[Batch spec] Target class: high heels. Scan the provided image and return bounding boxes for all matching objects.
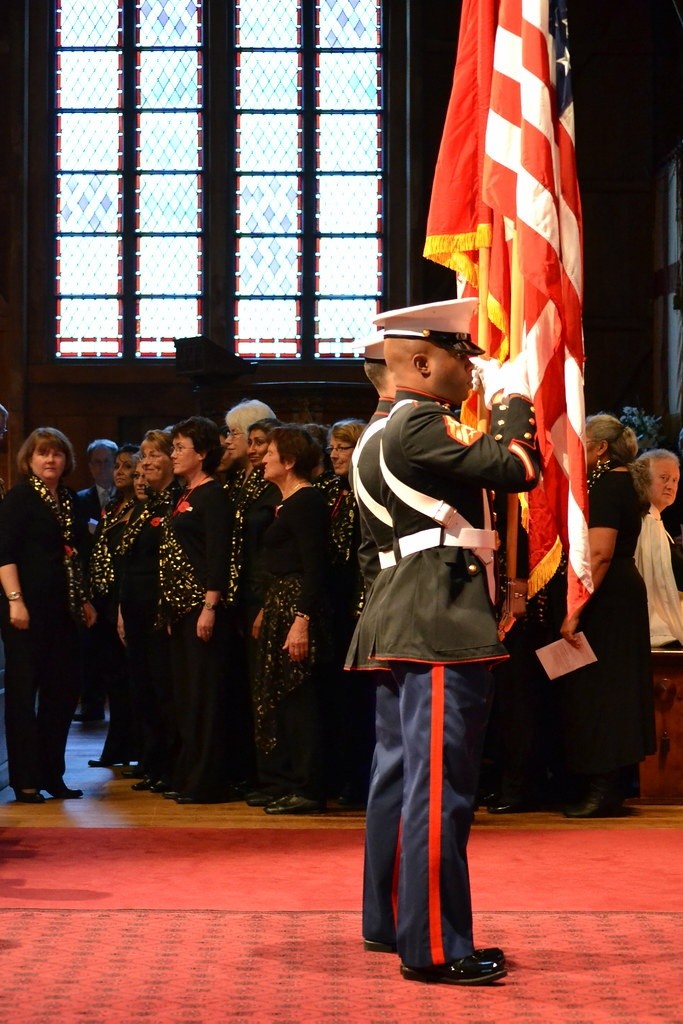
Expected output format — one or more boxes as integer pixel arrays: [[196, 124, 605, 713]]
[[88, 759, 130, 767], [12, 785, 44, 803], [38, 780, 83, 798]]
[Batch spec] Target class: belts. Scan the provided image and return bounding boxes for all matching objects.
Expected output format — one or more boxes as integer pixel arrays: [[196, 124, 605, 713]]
[[397, 527, 496, 559], [377, 549, 397, 570]]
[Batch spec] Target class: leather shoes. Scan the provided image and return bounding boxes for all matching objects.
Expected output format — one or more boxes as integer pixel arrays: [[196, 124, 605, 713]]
[[131, 774, 172, 794], [565, 780, 625, 818], [400, 948, 504, 975], [121, 764, 147, 778], [243, 789, 289, 806], [337, 783, 368, 807], [475, 785, 534, 815], [164, 786, 208, 804], [265, 793, 326, 815], [403, 954, 507, 985]]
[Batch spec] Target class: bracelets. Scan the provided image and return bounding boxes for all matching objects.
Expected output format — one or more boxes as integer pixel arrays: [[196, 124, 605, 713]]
[[295, 612, 309, 621], [514, 591, 526, 598]]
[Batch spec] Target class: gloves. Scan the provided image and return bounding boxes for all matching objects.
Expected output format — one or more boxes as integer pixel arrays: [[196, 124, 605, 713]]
[[469, 356, 504, 410], [499, 351, 535, 402]]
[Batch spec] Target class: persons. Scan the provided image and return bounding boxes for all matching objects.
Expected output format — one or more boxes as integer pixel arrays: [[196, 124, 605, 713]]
[[78, 398, 278, 803], [342, 296, 543, 985], [229, 418, 367, 812], [478, 409, 683, 819], [0, 426, 97, 802]]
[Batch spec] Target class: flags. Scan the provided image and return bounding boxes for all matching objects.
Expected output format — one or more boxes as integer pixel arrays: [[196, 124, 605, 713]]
[[423, 0, 594, 621]]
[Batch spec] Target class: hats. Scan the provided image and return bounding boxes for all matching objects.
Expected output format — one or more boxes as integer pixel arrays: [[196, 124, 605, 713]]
[[366, 330, 386, 360], [373, 295, 486, 355]]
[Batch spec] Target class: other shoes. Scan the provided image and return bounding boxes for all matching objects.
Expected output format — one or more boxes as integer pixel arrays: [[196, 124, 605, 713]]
[[225, 778, 257, 800], [73, 711, 105, 722], [363, 935, 397, 953]]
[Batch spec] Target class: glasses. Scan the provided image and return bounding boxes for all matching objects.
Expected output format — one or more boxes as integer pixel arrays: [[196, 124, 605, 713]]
[[0, 428, 8, 440], [226, 432, 246, 439], [170, 445, 195, 454], [326, 446, 354, 454]]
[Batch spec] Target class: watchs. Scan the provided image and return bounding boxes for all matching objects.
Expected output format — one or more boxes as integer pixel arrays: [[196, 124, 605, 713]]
[[204, 602, 216, 610], [7, 591, 23, 601]]
